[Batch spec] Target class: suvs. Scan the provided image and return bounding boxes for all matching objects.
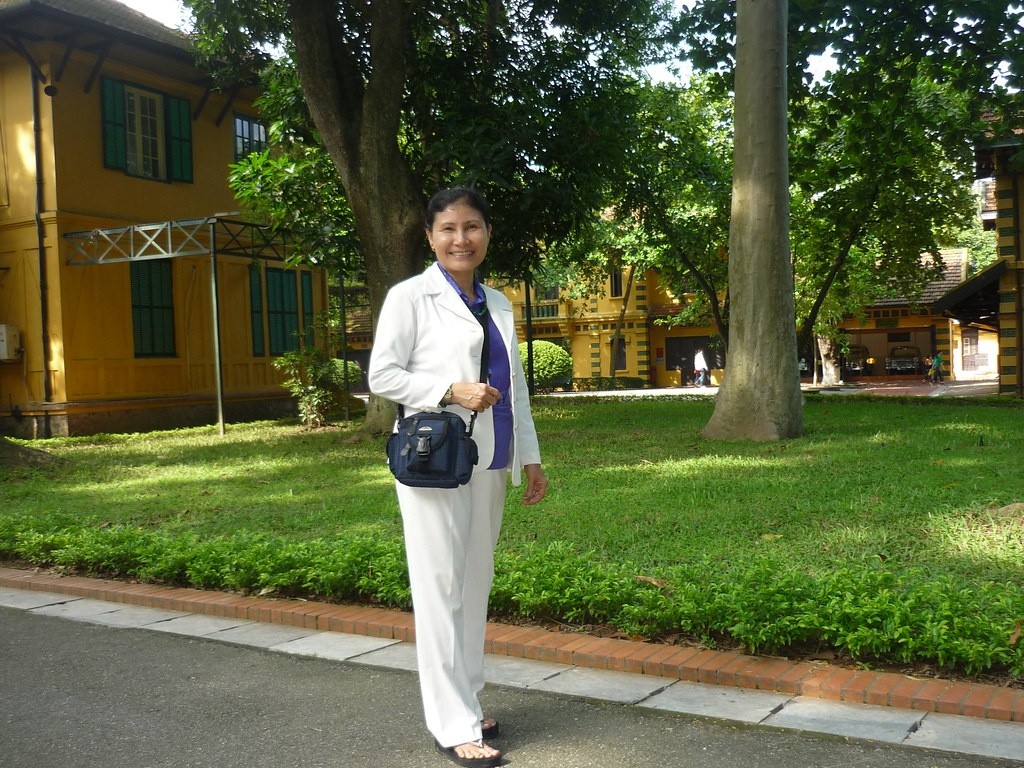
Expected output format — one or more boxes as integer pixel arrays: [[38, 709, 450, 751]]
[[886, 345, 923, 375], [839, 344, 873, 377]]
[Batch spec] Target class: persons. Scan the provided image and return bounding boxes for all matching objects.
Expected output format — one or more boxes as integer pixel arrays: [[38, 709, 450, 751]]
[[694, 348, 708, 387], [929, 351, 946, 384], [367, 189, 549, 768]]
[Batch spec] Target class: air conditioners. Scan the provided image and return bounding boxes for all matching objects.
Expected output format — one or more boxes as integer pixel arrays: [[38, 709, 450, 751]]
[[0, 323, 22, 360]]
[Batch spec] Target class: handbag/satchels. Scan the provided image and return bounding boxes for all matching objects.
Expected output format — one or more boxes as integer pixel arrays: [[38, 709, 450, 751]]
[[386, 411, 479, 488]]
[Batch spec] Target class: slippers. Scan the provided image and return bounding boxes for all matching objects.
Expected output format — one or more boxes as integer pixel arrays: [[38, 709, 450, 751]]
[[478, 713, 499, 740], [434, 734, 501, 767]]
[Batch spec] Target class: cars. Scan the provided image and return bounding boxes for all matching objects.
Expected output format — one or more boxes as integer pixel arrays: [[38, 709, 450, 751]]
[[797, 353, 815, 379]]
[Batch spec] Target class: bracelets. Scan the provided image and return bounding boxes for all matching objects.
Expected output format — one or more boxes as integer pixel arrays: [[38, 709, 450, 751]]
[[443, 387, 451, 405]]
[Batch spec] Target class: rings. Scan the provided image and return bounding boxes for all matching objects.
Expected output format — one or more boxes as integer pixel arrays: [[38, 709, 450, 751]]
[[533, 490, 537, 493]]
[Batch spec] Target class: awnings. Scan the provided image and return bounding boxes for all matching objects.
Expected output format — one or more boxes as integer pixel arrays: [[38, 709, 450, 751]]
[[929, 258, 1007, 331]]
